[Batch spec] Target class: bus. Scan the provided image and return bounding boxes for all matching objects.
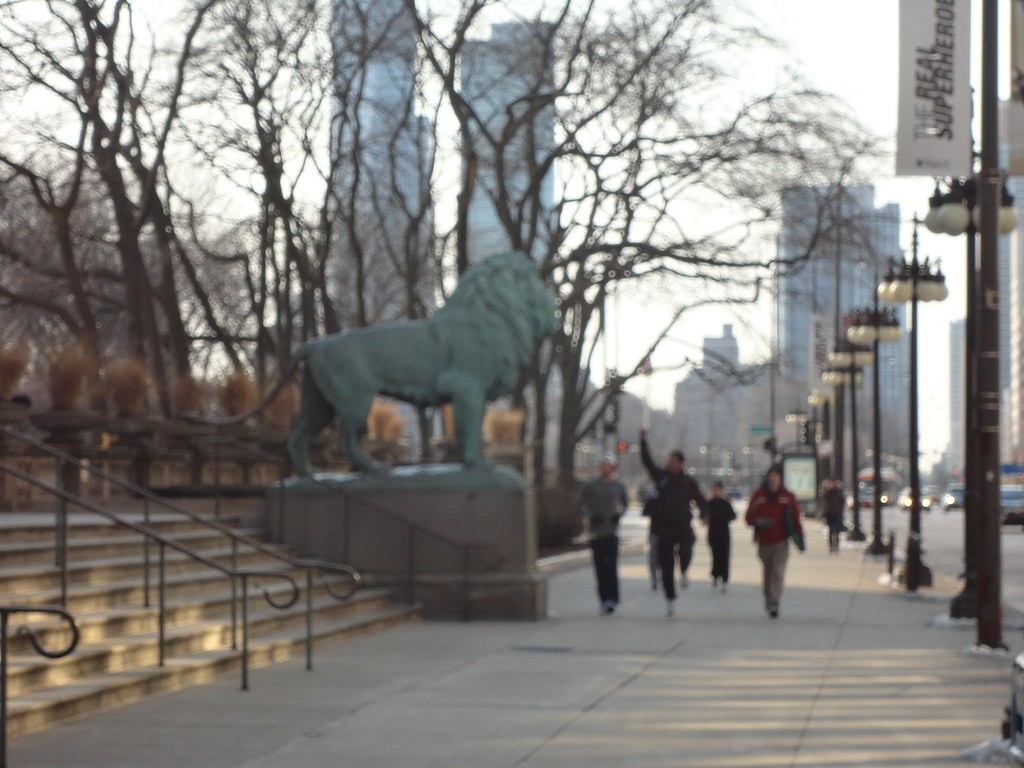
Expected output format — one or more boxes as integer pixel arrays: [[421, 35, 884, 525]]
[[857, 466, 903, 508]]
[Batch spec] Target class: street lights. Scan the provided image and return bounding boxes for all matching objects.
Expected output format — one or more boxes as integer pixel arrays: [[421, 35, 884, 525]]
[[922, 172, 1023, 618], [786, 335, 873, 536], [844, 281, 903, 557], [875, 210, 950, 591]]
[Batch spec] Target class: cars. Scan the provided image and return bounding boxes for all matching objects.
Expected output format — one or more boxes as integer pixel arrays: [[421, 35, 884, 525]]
[[942, 486, 967, 511], [998, 484, 1024, 526], [898, 485, 934, 512]]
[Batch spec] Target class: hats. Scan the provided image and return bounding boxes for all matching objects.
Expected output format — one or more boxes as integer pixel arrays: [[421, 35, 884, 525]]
[[599, 452, 619, 470]]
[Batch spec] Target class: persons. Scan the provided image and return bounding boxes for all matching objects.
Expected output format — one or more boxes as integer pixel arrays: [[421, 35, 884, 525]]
[[638, 429, 709, 602], [579, 452, 630, 613], [820, 478, 846, 553], [746, 466, 805, 617], [700, 482, 736, 593]]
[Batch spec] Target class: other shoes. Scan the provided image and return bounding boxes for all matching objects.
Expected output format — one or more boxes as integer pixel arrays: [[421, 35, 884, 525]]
[[603, 601, 615, 614], [768, 604, 778, 617]]
